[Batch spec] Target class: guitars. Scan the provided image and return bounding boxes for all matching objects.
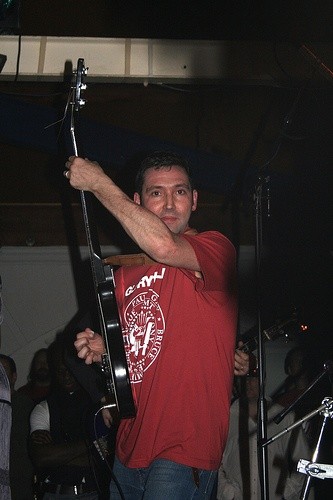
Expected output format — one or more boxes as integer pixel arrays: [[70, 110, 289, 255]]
[[69, 58, 136, 419], [94, 308, 301, 461]]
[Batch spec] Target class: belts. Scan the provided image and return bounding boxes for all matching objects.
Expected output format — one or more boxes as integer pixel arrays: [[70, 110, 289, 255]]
[[43, 483, 97, 495]]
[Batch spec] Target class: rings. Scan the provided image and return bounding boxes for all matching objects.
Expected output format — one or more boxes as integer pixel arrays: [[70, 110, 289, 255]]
[[64, 170, 70, 177]]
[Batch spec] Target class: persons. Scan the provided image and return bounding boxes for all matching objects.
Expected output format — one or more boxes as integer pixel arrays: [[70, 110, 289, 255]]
[[276, 348, 318, 407], [74, 327, 250, 377], [0, 344, 314, 500], [218, 364, 316, 500], [62, 152, 237, 500]]
